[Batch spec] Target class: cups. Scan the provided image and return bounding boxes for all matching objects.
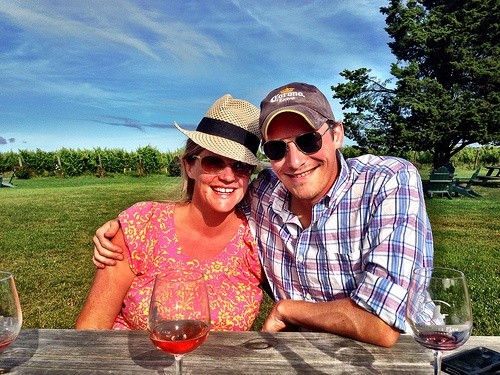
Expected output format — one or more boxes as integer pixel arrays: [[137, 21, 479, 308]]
[[0, 270, 22, 351]]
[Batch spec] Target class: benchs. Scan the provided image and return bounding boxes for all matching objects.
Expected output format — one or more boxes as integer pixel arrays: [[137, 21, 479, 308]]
[[478, 175, 500, 183]]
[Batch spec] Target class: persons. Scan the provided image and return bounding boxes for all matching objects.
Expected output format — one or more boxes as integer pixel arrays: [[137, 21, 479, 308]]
[[91, 83, 445, 348], [75, 93, 266, 334]]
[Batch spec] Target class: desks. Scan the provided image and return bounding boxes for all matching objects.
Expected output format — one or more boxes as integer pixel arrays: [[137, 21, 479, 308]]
[[0, 328, 500, 375], [484, 166, 500, 176]]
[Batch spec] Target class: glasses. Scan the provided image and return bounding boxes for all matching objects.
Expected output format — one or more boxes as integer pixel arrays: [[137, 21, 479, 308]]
[[191, 154, 256, 178], [263, 124, 334, 162]]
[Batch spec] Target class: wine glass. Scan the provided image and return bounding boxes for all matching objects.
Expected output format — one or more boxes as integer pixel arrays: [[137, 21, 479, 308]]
[[146, 269, 211, 375], [406, 267, 472, 375]]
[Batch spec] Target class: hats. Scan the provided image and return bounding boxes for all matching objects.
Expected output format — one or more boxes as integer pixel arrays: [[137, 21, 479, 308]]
[[259, 82, 335, 141], [172, 94, 263, 172]]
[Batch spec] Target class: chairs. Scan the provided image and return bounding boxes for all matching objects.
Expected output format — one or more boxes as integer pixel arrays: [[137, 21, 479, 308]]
[[454, 166, 482, 198], [428, 166, 452, 199]]
